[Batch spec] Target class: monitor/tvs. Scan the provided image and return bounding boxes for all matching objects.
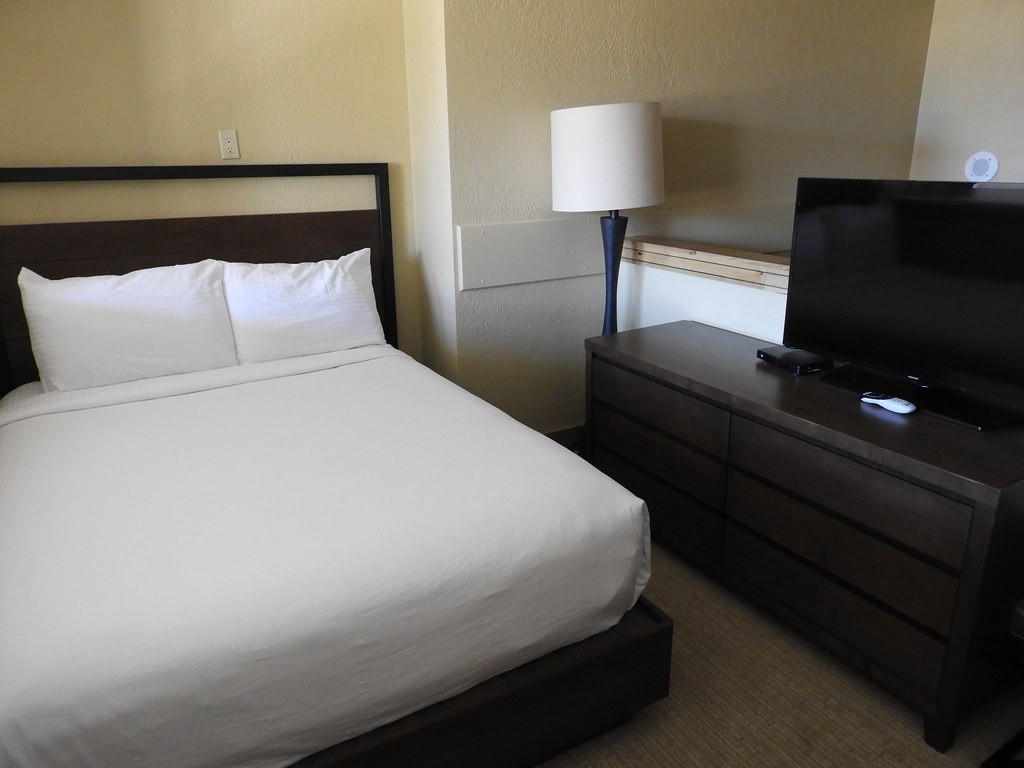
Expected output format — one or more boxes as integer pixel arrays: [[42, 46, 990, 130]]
[[782, 178, 1023, 429]]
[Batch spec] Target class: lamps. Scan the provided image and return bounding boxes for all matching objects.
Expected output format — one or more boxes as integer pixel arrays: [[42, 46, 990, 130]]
[[551, 102, 665, 335]]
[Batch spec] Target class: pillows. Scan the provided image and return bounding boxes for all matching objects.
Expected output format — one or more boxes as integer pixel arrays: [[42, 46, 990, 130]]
[[219, 248, 386, 366], [18, 258, 239, 393]]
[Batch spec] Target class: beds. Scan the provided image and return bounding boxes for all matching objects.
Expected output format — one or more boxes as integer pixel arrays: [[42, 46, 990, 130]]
[[1, 163, 674, 768]]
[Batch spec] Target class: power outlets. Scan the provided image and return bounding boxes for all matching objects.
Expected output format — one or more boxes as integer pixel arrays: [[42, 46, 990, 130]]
[[219, 130, 240, 160]]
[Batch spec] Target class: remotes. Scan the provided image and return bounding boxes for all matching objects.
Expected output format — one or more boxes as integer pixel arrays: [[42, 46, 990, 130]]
[[860, 390, 915, 414]]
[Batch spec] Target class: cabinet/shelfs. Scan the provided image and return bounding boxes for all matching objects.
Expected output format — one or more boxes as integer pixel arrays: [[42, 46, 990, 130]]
[[585, 320, 1024, 755]]
